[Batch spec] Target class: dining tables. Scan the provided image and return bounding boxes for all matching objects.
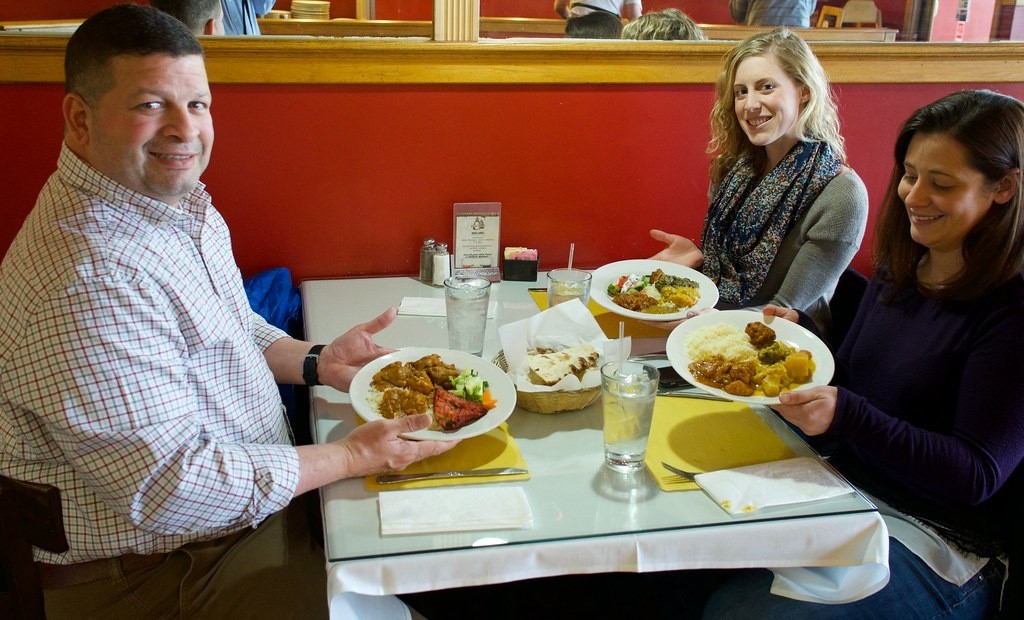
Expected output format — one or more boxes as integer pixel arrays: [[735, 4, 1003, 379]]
[[299, 269, 890, 620]]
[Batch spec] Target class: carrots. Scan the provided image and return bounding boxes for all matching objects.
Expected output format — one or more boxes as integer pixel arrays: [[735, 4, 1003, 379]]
[[481, 389, 497, 409]]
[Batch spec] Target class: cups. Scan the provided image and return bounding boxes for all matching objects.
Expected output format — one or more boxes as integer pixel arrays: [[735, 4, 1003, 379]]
[[547, 268, 593, 308], [443, 274, 491, 359], [600, 360, 660, 473]]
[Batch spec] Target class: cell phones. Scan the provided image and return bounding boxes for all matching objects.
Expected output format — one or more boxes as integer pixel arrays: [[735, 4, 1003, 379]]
[[656, 366, 691, 389]]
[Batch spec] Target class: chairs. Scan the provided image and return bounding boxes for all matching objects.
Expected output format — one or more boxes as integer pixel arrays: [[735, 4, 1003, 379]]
[[0, 475, 70, 620]]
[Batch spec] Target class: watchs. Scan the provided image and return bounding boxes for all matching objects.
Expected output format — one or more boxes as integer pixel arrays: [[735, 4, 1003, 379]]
[[303, 345, 326, 386]]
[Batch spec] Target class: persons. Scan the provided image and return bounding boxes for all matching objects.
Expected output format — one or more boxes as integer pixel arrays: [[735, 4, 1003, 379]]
[[221, 0, 276, 35], [621, 8, 706, 42], [565, 12, 623, 39], [149, 1, 225, 35], [0, 6, 464, 620], [730, 0, 817, 29], [555, 0, 643, 20], [705, 89, 1020, 619], [646, 26, 869, 310]]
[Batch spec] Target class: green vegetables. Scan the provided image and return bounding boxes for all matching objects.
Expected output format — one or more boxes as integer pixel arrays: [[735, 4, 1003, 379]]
[[448, 368, 489, 402]]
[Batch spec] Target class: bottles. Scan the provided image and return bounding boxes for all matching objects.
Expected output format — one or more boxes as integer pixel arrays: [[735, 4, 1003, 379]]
[[432, 242, 450, 288], [418, 237, 435, 281]]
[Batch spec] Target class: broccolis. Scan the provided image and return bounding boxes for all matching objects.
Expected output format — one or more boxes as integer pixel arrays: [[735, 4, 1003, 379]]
[[608, 276, 651, 294]]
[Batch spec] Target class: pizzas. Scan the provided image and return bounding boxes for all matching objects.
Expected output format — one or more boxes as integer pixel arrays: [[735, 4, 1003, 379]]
[[434, 388, 487, 428]]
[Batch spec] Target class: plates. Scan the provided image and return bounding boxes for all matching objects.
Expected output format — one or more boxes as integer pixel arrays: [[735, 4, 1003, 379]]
[[589, 259, 719, 321], [665, 310, 835, 405], [349, 347, 517, 443], [290, 0, 331, 20]]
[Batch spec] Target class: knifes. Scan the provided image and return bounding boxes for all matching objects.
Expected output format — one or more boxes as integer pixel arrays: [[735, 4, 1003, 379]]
[[377, 467, 528, 485], [656, 389, 734, 402], [529, 287, 547, 292]]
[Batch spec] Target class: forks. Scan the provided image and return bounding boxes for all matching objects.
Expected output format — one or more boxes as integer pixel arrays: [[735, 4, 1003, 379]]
[[660, 460, 704, 480]]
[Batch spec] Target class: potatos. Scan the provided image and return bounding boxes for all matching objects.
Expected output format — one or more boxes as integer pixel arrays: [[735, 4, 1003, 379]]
[[753, 352, 810, 395]]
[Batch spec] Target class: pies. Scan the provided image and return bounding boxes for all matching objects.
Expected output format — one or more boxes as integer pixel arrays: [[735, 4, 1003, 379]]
[[526, 343, 600, 385]]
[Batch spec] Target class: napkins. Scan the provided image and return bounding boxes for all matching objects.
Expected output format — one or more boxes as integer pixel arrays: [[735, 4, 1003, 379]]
[[378, 486, 534, 536], [694, 455, 856, 518], [398, 295, 497, 320]]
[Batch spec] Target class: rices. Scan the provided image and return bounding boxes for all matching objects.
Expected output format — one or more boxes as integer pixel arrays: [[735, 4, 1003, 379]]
[[685, 323, 757, 363], [644, 284, 662, 300]]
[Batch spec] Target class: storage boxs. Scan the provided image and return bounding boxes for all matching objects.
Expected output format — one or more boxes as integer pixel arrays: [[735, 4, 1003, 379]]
[[501, 254, 541, 283]]
